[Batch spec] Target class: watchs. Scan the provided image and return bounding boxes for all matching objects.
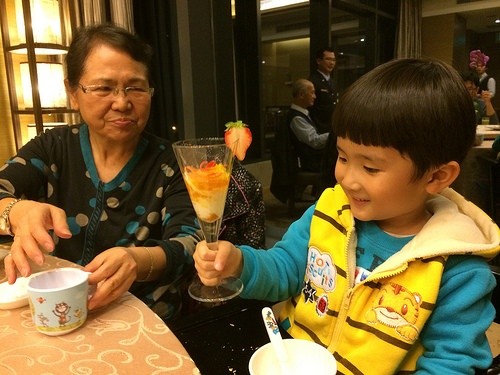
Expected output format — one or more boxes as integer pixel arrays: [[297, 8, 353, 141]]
[[0, 198, 23, 237]]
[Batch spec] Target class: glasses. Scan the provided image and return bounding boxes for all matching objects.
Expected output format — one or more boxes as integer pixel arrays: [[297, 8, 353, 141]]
[[76, 82, 154, 99]]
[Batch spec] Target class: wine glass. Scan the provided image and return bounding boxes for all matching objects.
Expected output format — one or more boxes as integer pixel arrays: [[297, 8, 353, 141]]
[[172, 138, 244, 302]]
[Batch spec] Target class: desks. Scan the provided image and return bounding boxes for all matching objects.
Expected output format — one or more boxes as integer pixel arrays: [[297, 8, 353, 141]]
[[449, 123, 500, 221], [0, 242, 202, 375]]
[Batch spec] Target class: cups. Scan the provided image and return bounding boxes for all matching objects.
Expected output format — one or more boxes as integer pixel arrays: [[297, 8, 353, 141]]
[[25, 267, 107, 336], [481, 117, 489, 125], [248, 339, 338, 375]]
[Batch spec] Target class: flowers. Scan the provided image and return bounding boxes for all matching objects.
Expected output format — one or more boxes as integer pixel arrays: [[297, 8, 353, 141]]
[[469, 48, 489, 72]]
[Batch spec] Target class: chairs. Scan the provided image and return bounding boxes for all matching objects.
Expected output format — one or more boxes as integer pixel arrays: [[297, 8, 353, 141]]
[[475, 152, 500, 223], [270, 111, 325, 218]]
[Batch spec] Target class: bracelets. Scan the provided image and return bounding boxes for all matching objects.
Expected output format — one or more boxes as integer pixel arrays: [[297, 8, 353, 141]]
[[136, 246, 153, 283]]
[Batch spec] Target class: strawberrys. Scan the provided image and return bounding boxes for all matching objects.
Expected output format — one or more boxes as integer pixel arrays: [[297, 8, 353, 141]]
[[224, 120, 252, 161]]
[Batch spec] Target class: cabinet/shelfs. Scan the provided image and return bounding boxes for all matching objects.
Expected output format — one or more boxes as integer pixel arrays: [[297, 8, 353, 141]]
[[0, 0, 81, 154]]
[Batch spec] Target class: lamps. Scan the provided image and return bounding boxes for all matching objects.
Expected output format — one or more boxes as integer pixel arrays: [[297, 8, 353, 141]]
[[4, 0, 69, 55], [28, 123, 68, 143], [19, 61, 68, 109]]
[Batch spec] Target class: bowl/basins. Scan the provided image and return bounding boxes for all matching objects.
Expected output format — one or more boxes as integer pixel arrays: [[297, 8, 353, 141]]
[[474, 133, 484, 146]]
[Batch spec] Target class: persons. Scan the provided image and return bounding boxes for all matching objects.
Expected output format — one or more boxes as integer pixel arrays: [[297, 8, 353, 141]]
[[284, 78, 332, 174], [194, 57, 500, 375], [461, 50, 499, 124], [0, 25, 213, 332], [309, 47, 340, 135]]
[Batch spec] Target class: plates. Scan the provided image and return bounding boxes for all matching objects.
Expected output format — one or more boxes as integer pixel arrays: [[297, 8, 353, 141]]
[[0, 277, 29, 310], [476, 124, 500, 139]]
